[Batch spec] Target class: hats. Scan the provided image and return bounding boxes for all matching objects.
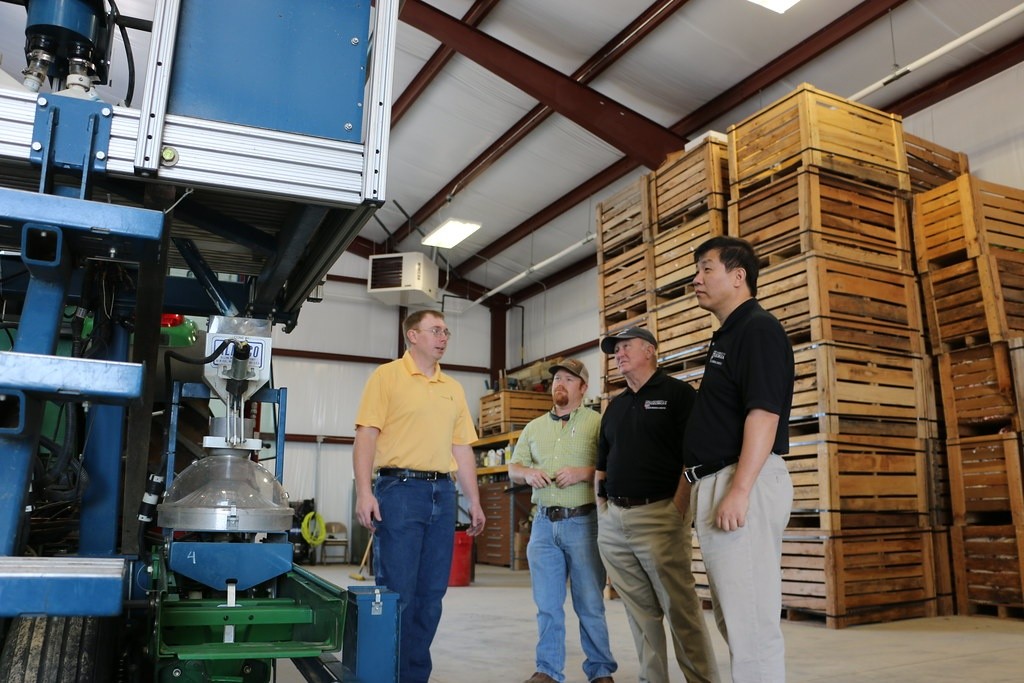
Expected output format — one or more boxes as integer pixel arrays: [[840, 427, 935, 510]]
[[547, 359, 591, 384], [600, 327, 659, 351]]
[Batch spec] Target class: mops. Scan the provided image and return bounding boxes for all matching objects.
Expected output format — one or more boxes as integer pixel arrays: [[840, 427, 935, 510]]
[[349, 532, 374, 581]]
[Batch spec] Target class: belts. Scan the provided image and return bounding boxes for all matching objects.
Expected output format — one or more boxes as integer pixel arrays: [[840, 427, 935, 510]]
[[541, 503, 596, 521], [378, 467, 449, 480], [681, 457, 742, 484], [607, 496, 669, 510]]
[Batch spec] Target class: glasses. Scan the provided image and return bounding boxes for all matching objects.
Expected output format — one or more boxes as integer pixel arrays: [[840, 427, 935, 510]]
[[414, 329, 453, 340], [548, 412, 574, 422]]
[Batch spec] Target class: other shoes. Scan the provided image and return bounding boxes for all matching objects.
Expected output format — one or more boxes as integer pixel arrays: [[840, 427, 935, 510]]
[[527, 671, 560, 683], [590, 676, 614, 683]]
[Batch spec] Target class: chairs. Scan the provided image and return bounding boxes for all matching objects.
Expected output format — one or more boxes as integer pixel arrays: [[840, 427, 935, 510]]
[[320, 522, 350, 567]]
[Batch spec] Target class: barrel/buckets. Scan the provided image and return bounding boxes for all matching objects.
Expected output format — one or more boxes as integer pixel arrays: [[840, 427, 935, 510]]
[[447, 532, 472, 586]]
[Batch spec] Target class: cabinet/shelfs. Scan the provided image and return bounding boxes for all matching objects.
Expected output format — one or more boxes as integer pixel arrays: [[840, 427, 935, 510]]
[[470, 429, 534, 571]]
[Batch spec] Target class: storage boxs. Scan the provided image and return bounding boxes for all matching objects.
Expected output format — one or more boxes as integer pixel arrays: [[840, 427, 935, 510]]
[[513, 531, 529, 571], [597, 81, 1024, 630], [479, 390, 554, 438]]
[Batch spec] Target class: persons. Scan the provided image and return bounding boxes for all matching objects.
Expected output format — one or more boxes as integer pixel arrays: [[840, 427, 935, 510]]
[[508, 357, 619, 683], [594, 325, 722, 683], [681, 237, 794, 683], [352, 309, 487, 683]]
